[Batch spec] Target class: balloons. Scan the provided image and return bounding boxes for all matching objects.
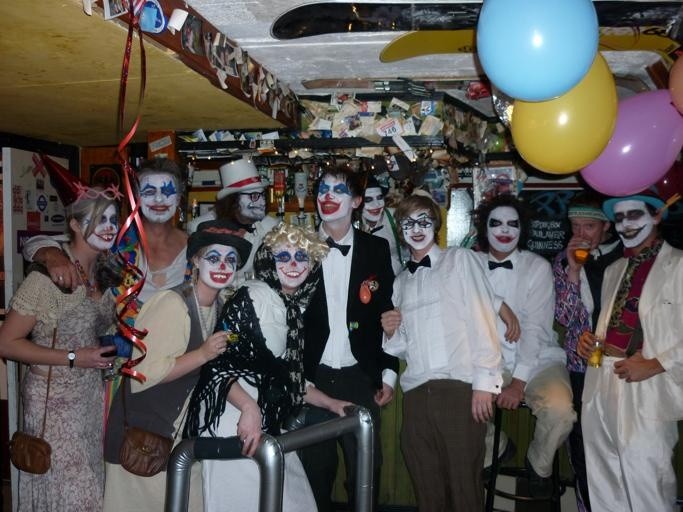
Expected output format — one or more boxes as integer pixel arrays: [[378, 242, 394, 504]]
[[576, 88, 682, 198], [474, 1, 599, 104], [669, 54, 682, 116], [509, 51, 618, 177]]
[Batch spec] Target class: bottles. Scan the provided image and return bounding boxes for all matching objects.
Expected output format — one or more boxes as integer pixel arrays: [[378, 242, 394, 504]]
[[294, 168, 309, 221], [257, 164, 269, 195], [272, 168, 288, 219]]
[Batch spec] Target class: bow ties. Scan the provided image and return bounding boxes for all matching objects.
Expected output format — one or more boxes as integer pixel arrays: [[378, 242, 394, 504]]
[[488, 260, 512, 270], [406, 255, 431, 274], [326, 236, 350, 256]]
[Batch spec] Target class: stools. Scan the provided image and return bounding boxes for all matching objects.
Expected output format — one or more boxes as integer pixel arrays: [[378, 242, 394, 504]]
[[483, 402, 581, 512]]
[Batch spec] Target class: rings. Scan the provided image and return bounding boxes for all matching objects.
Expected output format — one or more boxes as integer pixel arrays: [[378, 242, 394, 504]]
[[58, 275, 63, 281]]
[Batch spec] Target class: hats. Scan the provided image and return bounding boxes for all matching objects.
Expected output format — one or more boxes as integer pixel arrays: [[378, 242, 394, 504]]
[[358, 171, 389, 198], [602, 184, 668, 223], [216, 158, 270, 200], [36, 151, 88, 208], [186, 220, 252, 271]]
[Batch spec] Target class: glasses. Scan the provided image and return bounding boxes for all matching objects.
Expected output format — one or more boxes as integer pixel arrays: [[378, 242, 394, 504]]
[[243, 190, 270, 202], [73, 182, 122, 202], [399, 217, 431, 230]]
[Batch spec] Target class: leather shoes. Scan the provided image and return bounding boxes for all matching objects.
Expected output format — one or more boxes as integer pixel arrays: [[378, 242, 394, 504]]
[[482, 437, 515, 482], [524, 454, 553, 500]]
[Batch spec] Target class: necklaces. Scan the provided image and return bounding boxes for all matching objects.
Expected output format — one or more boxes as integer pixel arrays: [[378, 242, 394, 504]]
[[190, 288, 217, 339]]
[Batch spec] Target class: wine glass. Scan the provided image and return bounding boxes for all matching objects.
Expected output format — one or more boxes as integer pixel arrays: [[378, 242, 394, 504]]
[[97, 334, 123, 383]]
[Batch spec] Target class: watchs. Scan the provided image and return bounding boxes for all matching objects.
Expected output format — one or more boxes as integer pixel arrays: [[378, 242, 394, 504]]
[[67, 350, 75, 369]]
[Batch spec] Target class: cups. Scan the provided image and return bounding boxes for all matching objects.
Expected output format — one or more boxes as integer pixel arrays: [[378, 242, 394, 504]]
[[572, 237, 590, 265], [587, 331, 605, 369]]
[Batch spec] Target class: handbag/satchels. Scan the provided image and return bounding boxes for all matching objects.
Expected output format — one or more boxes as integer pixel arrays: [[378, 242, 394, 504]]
[[10, 432, 51, 474], [120, 429, 174, 476]]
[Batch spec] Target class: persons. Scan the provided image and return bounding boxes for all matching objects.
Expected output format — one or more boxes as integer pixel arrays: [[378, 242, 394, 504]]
[[102, 218, 252, 511], [379, 189, 503, 512], [171, 221, 356, 512], [465, 193, 578, 501], [352, 174, 412, 276], [180, 159, 280, 286], [19, 157, 190, 431], [0, 186, 123, 512], [294, 165, 399, 512], [552, 188, 624, 512], [581, 183, 683, 512]]
